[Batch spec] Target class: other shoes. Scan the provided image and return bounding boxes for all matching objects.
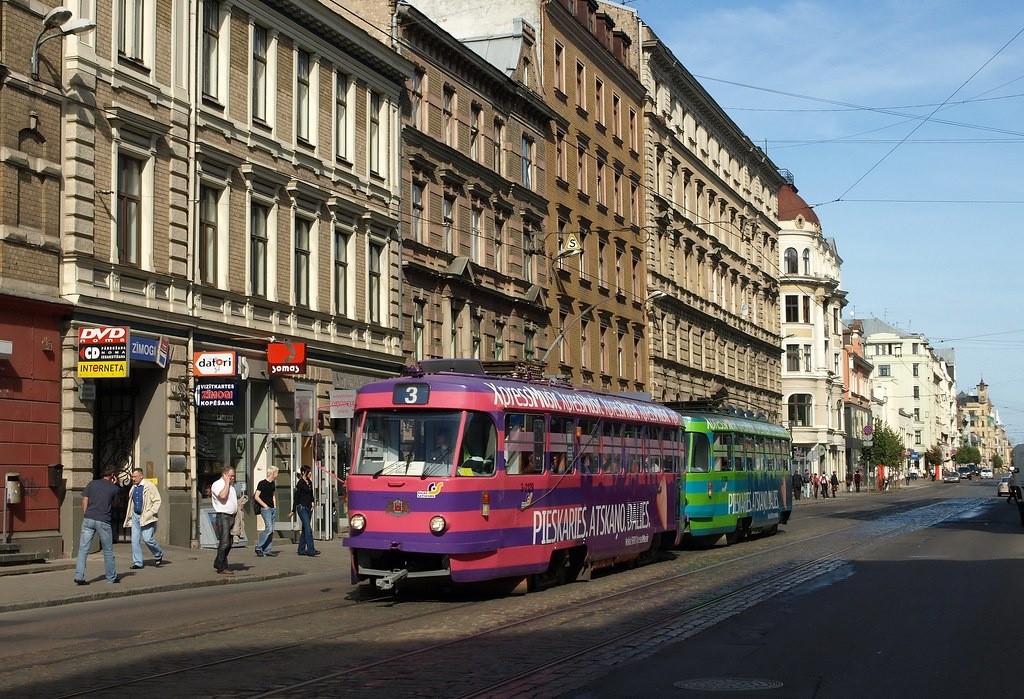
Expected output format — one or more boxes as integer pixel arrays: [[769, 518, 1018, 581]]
[[113, 579, 120, 583], [74, 579, 89, 585], [155, 557, 162, 566], [130, 565, 144, 569], [298, 550, 321, 556], [214, 565, 235, 575], [255, 549, 277, 558]]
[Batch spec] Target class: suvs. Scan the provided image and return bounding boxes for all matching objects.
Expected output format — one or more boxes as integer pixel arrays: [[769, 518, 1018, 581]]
[[957, 467, 973, 481]]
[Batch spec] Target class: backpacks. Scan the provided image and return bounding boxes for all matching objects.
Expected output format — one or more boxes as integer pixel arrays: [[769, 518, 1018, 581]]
[[821, 476, 827, 485]]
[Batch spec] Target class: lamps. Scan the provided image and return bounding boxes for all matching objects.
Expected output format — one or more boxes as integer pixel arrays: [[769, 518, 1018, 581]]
[[642, 291, 662, 311], [36, 18, 98, 74], [33, 6, 72, 74], [653, 291, 667, 306], [780, 332, 797, 341]]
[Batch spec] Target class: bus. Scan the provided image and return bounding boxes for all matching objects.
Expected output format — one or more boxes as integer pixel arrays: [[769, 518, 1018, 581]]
[[341, 358, 685, 598], [658, 399, 795, 546]]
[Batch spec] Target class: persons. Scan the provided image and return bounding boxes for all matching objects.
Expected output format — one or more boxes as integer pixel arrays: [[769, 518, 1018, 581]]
[[846, 470, 861, 492], [74, 465, 120, 585], [426, 426, 474, 477], [287, 464, 321, 556], [792, 469, 839, 500], [1007, 471, 1019, 505], [583, 453, 659, 473], [211, 466, 238, 574], [255, 466, 279, 557], [123, 468, 163, 569], [889, 465, 934, 487]]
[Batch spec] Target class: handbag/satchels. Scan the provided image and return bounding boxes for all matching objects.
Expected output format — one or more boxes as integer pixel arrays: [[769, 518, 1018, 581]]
[[252, 493, 261, 515]]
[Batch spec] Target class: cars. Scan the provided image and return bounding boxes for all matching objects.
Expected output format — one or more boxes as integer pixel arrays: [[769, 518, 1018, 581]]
[[944, 472, 961, 483], [980, 469, 994, 479], [997, 476, 1017, 498]]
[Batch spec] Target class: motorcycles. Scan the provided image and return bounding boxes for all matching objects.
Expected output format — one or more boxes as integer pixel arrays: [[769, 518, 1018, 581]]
[[975, 469, 979, 476]]
